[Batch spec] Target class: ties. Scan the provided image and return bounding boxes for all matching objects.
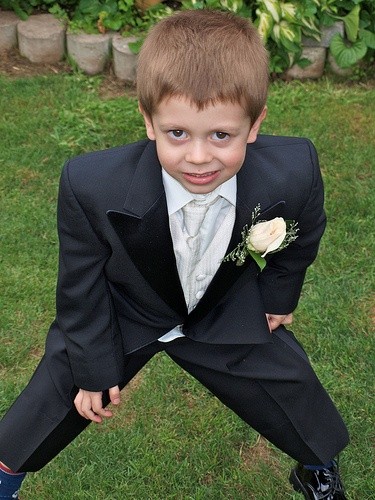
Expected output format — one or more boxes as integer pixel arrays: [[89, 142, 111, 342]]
[[181, 200, 207, 258]]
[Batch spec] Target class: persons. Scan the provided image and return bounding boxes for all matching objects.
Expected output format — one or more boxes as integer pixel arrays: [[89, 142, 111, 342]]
[[0, 7, 350, 500]]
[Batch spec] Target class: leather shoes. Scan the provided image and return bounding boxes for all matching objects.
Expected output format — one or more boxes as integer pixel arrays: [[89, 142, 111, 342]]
[[289, 465, 348, 499]]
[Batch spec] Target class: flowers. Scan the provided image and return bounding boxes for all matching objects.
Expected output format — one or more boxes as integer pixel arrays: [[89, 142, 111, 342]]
[[217, 202, 299, 272]]
